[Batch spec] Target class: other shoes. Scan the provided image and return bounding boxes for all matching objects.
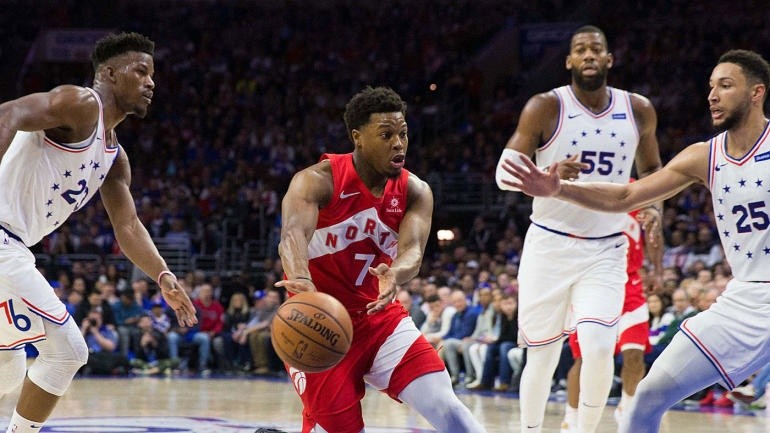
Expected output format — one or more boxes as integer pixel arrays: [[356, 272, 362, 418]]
[[129, 360, 285, 378], [452, 373, 770, 433]]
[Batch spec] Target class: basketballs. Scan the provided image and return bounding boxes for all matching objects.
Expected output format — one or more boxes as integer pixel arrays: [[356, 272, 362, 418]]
[[271, 292, 353, 373]]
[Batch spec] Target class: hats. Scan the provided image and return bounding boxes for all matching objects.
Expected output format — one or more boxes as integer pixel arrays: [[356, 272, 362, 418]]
[[151, 300, 164, 307], [467, 261, 478, 269], [252, 290, 266, 299]]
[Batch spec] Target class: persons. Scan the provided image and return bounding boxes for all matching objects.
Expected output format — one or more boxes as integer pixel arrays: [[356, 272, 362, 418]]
[[495, 46, 770, 433], [0, 30, 202, 433], [558, 176, 653, 433], [250, 84, 491, 433], [156, 271, 177, 289], [490, 22, 668, 433], [0, 0, 770, 410]]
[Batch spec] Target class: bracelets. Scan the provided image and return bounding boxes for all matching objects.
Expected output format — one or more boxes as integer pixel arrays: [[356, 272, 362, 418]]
[[290, 275, 314, 281]]
